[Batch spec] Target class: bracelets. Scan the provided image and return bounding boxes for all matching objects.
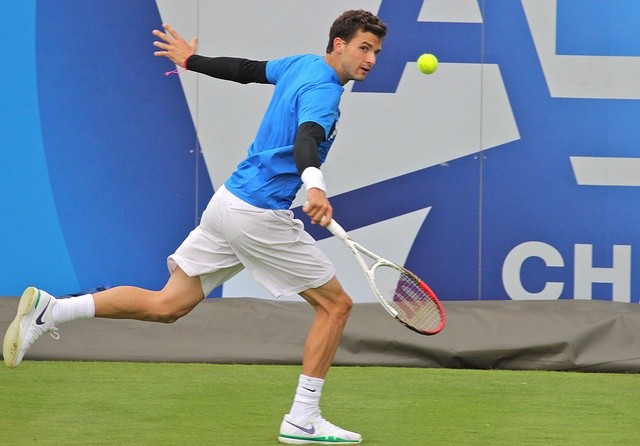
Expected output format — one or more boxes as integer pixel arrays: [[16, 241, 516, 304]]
[[184, 54, 194, 70]]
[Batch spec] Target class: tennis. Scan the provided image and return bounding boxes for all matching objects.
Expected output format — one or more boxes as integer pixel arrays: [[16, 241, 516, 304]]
[[416, 53, 438, 74]]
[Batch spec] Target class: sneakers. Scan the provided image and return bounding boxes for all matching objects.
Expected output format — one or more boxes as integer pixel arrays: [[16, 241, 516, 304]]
[[278, 413, 363, 445], [2, 286, 60, 369]]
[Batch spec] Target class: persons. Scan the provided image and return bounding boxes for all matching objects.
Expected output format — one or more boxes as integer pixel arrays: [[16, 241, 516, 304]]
[[2, 10, 386, 445]]
